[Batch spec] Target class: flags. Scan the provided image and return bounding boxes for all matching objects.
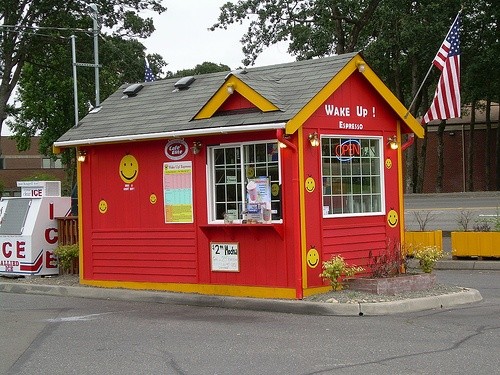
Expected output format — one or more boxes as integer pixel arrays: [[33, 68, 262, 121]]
[[144, 65, 156, 82], [419, 12, 462, 124]]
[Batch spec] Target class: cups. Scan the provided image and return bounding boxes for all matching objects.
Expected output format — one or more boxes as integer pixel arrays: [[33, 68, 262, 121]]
[[247, 181, 257, 201]]
